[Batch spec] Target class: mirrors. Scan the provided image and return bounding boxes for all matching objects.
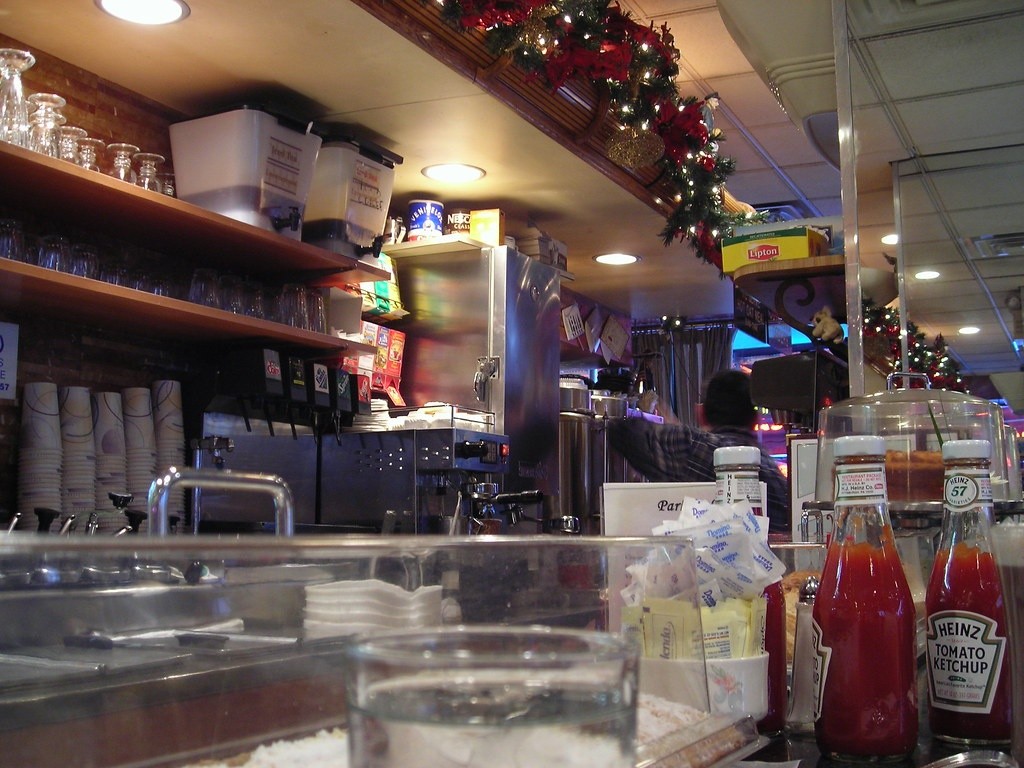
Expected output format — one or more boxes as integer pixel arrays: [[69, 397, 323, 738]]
[[830, 1, 1023, 502]]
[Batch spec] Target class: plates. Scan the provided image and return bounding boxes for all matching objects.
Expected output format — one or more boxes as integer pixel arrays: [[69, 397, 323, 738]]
[[301, 577, 443, 642]]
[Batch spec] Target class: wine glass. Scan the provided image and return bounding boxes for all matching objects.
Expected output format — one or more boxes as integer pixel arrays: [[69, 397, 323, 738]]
[[0, 47, 176, 198]]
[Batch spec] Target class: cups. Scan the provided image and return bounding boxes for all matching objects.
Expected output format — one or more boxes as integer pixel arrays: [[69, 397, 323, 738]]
[[504, 235, 519, 251], [0, 215, 327, 335], [469, 518, 503, 534], [15, 379, 186, 538], [338, 625, 643, 768], [987, 519, 1024, 768]]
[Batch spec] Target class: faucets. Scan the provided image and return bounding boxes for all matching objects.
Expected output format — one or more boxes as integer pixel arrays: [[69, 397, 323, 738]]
[[191, 435, 235, 534]]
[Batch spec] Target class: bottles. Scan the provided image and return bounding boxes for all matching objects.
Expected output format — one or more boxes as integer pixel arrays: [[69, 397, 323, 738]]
[[370, 509, 420, 592], [785, 600, 818, 744], [711, 444, 788, 730], [383, 215, 408, 246], [811, 433, 921, 767], [924, 438, 1012, 753]]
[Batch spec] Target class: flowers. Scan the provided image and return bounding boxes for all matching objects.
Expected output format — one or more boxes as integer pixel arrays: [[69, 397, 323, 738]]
[[429, 1, 976, 394]]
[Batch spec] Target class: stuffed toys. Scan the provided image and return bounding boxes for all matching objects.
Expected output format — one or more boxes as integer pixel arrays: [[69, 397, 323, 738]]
[[811, 307, 844, 344]]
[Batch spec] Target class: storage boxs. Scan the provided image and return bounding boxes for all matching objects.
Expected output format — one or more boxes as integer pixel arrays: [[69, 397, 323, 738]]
[[720, 225, 829, 276], [469, 208, 505, 246]]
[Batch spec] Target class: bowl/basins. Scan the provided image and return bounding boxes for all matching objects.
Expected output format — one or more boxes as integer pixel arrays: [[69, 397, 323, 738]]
[[645, 650, 770, 727]]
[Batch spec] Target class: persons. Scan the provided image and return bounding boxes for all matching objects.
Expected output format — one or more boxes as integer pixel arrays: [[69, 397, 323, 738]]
[[608, 370, 788, 533], [701, 91, 722, 132]]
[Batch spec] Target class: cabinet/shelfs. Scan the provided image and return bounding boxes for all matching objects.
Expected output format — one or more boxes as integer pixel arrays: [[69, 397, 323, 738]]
[[0, 140, 393, 360]]
